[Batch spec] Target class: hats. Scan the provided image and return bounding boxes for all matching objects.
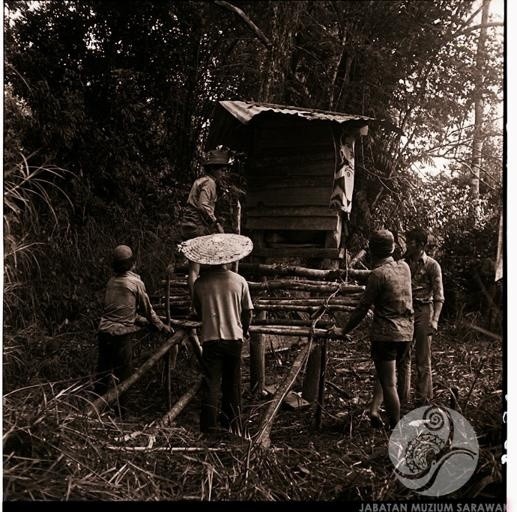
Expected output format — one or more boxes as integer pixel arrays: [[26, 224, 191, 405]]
[[202, 151, 231, 166], [179, 233, 256, 266], [113, 245, 132, 262], [370, 229, 397, 247]]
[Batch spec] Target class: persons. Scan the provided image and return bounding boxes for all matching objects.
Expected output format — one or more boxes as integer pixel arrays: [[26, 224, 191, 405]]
[[403, 228, 445, 407], [177, 233, 255, 433], [94, 244, 175, 399], [327, 230, 414, 429], [181, 151, 231, 297]]
